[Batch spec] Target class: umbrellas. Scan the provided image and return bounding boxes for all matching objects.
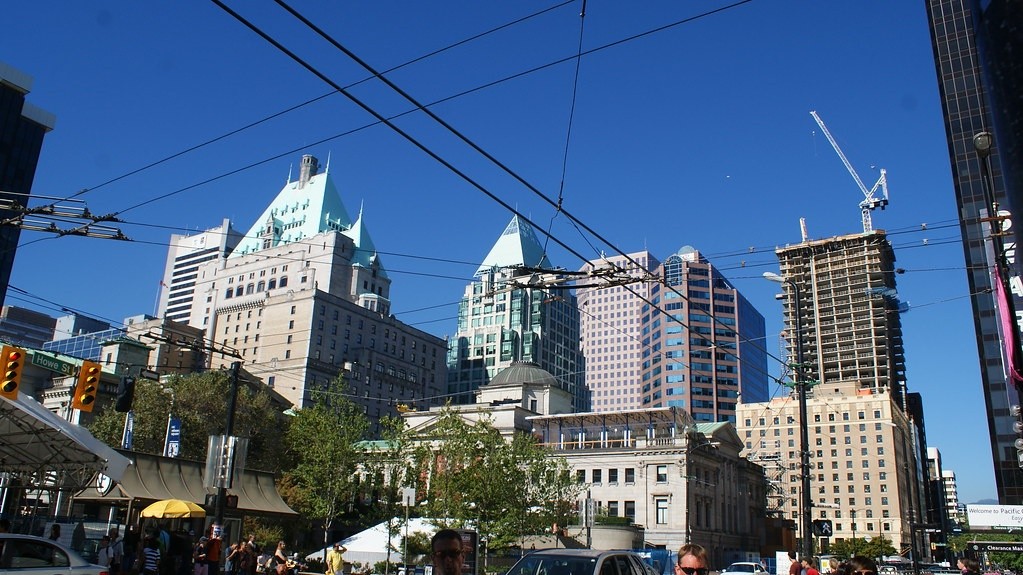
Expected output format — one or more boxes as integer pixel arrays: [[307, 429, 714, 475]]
[[140, 499, 206, 531]]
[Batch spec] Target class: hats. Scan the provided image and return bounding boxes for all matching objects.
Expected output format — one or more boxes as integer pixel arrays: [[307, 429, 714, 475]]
[[199, 536, 209, 543]]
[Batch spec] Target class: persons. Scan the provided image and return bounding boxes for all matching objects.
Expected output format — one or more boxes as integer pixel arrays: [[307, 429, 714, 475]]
[[759, 549, 880, 575], [431, 529, 466, 575], [0, 518, 14, 568], [49, 524, 61, 559], [97, 525, 302, 575], [326, 541, 347, 575], [674, 542, 711, 575], [956, 557, 981, 575]]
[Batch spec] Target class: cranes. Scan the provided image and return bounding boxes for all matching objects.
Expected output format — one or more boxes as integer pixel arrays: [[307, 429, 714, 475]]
[[810, 111, 889, 232]]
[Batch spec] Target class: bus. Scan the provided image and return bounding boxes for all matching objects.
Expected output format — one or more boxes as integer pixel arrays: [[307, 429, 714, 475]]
[[883, 556, 914, 575]]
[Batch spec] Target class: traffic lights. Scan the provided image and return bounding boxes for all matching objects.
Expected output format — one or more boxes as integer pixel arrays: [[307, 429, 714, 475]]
[[205, 494, 218, 507], [72, 361, 102, 412], [814, 520, 832, 536], [226, 494, 239, 509], [0, 345, 26, 400]]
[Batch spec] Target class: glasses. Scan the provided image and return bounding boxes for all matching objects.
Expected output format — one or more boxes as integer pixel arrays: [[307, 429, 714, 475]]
[[678, 564, 709, 575], [850, 570, 875, 575], [432, 549, 464, 558]]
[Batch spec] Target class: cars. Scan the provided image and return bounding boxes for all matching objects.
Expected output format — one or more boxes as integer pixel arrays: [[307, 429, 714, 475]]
[[505, 548, 649, 575], [928, 566, 943, 573], [0, 533, 110, 575], [719, 562, 770, 575]]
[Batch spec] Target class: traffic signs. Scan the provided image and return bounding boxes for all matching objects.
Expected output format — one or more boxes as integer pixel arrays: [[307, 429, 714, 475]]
[[32, 351, 74, 376]]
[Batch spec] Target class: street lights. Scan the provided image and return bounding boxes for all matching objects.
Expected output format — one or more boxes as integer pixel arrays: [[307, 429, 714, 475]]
[[762, 272, 813, 558], [881, 421, 920, 575], [852, 508, 870, 557], [685, 442, 721, 544], [879, 519, 895, 566]]
